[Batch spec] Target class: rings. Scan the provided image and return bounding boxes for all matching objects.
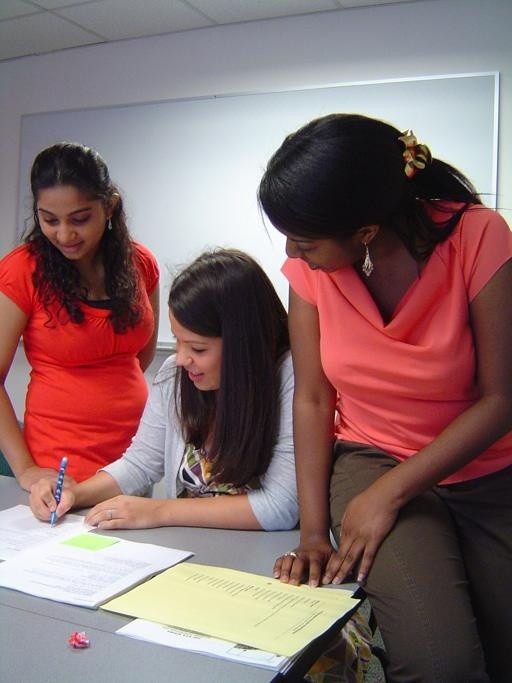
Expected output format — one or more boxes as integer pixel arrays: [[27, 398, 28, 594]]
[[284, 551, 297, 557], [108, 509, 113, 520]]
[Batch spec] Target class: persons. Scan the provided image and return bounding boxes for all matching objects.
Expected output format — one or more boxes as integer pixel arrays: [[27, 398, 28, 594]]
[[28, 249, 299, 531], [0, 141, 159, 492], [256, 114, 512, 683]]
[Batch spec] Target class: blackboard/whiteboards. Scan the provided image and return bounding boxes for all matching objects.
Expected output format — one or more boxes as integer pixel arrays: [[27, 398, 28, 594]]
[[17, 70, 503, 353]]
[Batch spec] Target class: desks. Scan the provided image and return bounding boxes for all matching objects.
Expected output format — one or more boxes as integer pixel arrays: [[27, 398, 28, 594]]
[[0, 473, 369, 682]]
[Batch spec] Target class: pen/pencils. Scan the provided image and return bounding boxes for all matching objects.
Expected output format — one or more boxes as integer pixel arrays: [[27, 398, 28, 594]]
[[49, 454, 68, 527]]
